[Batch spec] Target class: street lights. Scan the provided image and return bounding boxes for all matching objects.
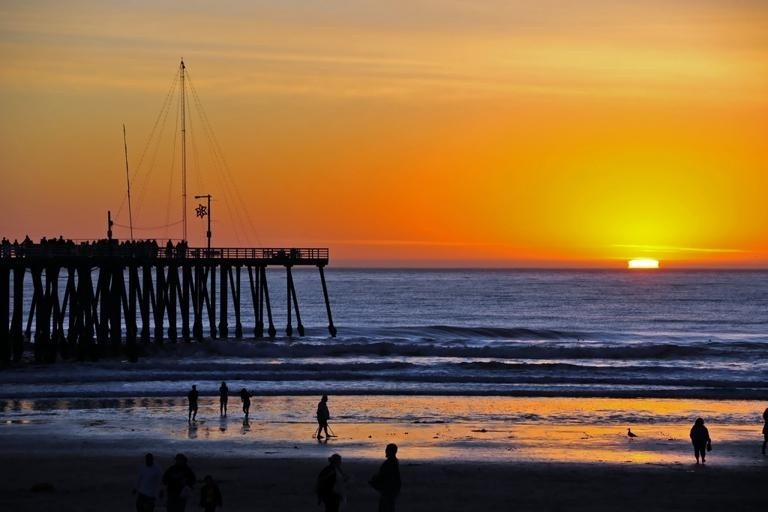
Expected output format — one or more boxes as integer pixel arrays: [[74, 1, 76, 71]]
[[195, 195, 210, 247]]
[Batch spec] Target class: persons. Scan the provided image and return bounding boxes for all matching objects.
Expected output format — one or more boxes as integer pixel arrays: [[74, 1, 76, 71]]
[[315, 453, 351, 510], [130, 452, 163, 512], [239, 414, 251, 435], [689, 417, 712, 465], [186, 384, 199, 423], [161, 453, 196, 512], [198, 473, 225, 512], [218, 380, 230, 419], [239, 387, 254, 417], [187, 420, 198, 439], [762, 407, 768, 455], [370, 443, 403, 512], [316, 394, 331, 439], [0, 233, 189, 260], [218, 414, 228, 433]]
